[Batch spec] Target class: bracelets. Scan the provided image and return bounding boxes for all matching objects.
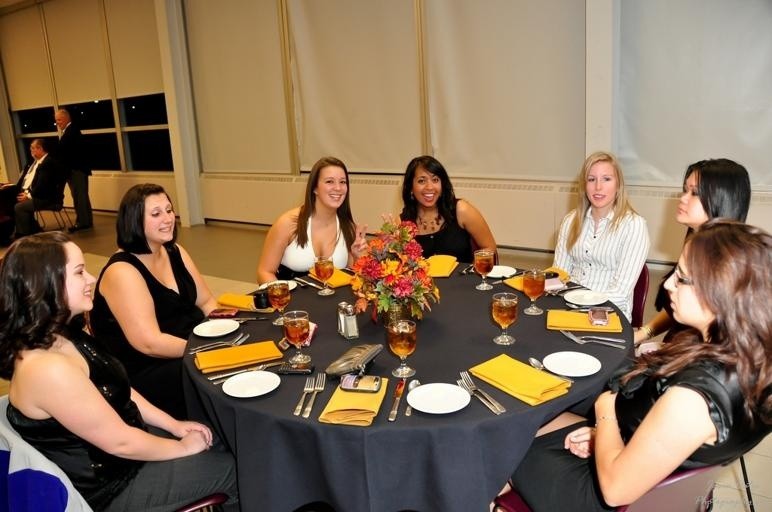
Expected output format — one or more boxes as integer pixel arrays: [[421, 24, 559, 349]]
[[640, 323, 654, 340], [591, 413, 619, 431]]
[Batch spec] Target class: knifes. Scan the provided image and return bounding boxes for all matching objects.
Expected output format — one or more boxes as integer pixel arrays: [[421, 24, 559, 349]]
[[387, 378, 407, 421], [208, 361, 284, 381], [491, 270, 525, 285], [294, 276, 323, 290]]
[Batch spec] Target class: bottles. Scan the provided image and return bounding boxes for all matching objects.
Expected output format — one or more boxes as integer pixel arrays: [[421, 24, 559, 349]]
[[337, 301, 347, 336], [345, 303, 360, 339]]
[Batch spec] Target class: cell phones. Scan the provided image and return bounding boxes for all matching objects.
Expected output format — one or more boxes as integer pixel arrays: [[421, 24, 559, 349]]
[[280, 361, 314, 375], [589, 309, 609, 326], [341, 375, 382, 392]]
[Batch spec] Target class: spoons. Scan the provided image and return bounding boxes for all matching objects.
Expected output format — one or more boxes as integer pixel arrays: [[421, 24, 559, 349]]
[[566, 303, 611, 309], [212, 364, 270, 386], [528, 357, 574, 383], [405, 379, 421, 417]]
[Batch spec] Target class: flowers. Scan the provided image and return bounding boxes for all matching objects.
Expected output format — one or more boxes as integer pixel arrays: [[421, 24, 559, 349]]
[[345, 220, 441, 324]]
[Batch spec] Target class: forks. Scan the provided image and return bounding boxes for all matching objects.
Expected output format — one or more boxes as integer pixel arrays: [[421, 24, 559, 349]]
[[294, 377, 315, 416], [567, 331, 626, 350], [189, 333, 244, 350], [460, 371, 507, 413], [188, 334, 250, 354], [302, 373, 326, 418], [456, 379, 501, 415], [560, 329, 626, 349]]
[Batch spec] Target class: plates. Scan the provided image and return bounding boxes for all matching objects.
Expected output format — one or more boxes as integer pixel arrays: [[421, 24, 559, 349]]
[[193, 318, 240, 338], [542, 351, 602, 377], [221, 371, 281, 398], [406, 382, 471, 414], [258, 279, 297, 291], [564, 290, 609, 306], [486, 265, 517, 278]]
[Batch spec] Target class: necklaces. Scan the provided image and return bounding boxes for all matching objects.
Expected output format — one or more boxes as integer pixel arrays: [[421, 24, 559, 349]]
[[417, 210, 443, 233]]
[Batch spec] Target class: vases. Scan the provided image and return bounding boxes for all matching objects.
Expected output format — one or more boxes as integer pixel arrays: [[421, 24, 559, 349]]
[[380, 298, 412, 331]]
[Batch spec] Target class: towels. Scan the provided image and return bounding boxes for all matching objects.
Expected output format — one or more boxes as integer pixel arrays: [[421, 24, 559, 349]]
[[216, 293, 276, 314], [503, 267, 569, 292], [194, 341, 284, 374], [308, 267, 351, 288], [468, 353, 572, 407], [546, 310, 623, 333], [426, 255, 459, 278], [318, 377, 389, 426]]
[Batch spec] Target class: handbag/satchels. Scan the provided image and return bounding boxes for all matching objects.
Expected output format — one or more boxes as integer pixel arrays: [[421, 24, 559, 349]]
[[324, 343, 384, 379]]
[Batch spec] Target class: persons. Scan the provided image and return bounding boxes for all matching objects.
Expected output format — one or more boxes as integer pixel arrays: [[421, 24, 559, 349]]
[[633, 158, 751, 358], [1, 231, 240, 511], [552, 151, 649, 324], [0, 138, 65, 247], [393, 155, 496, 263], [54, 108, 95, 232], [489, 217, 772, 512], [88, 182, 220, 421], [256, 156, 370, 285]]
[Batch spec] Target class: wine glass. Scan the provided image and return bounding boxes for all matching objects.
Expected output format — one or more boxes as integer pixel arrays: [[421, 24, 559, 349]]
[[523, 268, 544, 315], [267, 280, 292, 325], [314, 256, 335, 296], [282, 310, 311, 364], [387, 313, 417, 378], [491, 292, 518, 345], [474, 249, 495, 290]]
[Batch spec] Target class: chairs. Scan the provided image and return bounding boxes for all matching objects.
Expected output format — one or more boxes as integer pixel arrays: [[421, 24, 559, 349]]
[[631, 265, 649, 345], [31, 208, 74, 233], [494, 463, 727, 511], [0, 395, 230, 512], [721, 437, 767, 512]]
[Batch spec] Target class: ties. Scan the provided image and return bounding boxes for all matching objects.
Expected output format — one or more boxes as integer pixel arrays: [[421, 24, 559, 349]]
[[21, 160, 37, 188]]
